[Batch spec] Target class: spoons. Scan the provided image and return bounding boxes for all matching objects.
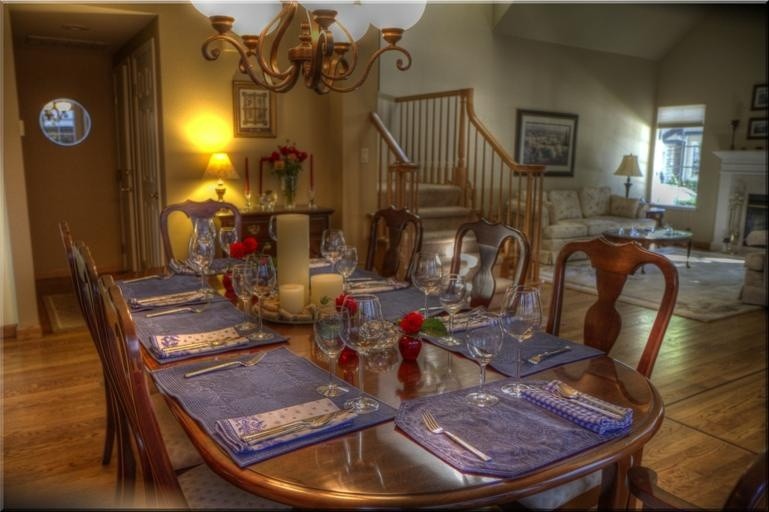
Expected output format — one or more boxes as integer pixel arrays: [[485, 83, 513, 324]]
[[245, 415, 330, 446], [555, 380, 627, 420]]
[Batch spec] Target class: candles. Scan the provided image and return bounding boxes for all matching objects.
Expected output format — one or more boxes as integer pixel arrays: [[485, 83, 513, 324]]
[[310, 154, 314, 189], [259, 156, 262, 194], [245, 157, 250, 194]]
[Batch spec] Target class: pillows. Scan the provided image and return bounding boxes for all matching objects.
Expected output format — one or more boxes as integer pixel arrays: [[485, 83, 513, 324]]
[[521, 187, 612, 223]]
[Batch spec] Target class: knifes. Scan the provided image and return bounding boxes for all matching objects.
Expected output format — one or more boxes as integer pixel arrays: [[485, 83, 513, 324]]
[[235, 404, 353, 442]]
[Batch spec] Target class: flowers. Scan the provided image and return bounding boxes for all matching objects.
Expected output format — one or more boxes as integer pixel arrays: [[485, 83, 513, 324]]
[[261, 139, 308, 175]]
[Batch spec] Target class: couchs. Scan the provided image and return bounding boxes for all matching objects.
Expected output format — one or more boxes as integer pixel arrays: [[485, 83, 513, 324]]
[[505, 186, 648, 269]]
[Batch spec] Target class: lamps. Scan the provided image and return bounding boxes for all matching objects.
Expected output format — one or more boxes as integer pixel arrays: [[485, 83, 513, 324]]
[[614, 153, 644, 197], [46, 103, 71, 120], [200, 150, 239, 202], [188, 1, 428, 94]]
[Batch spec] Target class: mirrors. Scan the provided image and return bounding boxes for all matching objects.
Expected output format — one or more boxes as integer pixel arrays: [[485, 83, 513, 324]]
[[39, 99, 92, 146]]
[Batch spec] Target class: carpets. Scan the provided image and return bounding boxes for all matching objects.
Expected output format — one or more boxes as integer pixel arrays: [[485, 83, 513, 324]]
[[45, 290, 87, 334]]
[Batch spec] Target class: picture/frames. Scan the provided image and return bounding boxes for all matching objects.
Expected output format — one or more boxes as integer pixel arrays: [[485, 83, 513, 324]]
[[746, 117, 766, 139], [513, 108, 579, 177], [750, 82, 767, 110], [232, 79, 277, 138]]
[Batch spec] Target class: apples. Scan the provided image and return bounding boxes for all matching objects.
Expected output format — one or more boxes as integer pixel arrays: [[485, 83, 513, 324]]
[[339, 348, 358, 370], [398, 336, 422, 361], [223, 270, 240, 290]]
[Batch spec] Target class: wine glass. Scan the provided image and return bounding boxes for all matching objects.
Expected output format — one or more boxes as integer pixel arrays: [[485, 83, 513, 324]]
[[316, 308, 350, 397], [185, 213, 239, 289], [320, 227, 359, 284], [340, 296, 386, 414], [438, 276, 466, 345], [233, 254, 276, 343], [464, 314, 503, 408], [499, 284, 538, 397], [412, 252, 441, 320]]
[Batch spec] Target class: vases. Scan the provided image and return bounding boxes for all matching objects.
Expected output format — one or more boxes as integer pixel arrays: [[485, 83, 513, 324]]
[[280, 175, 297, 208]]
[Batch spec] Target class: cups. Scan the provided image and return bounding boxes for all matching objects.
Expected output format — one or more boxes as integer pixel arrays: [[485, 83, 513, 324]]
[[258, 189, 278, 213]]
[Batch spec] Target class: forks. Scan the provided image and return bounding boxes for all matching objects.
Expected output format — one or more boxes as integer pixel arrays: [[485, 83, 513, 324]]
[[517, 344, 570, 367], [419, 411, 494, 466], [121, 271, 177, 284], [144, 298, 212, 318], [180, 349, 268, 379]]
